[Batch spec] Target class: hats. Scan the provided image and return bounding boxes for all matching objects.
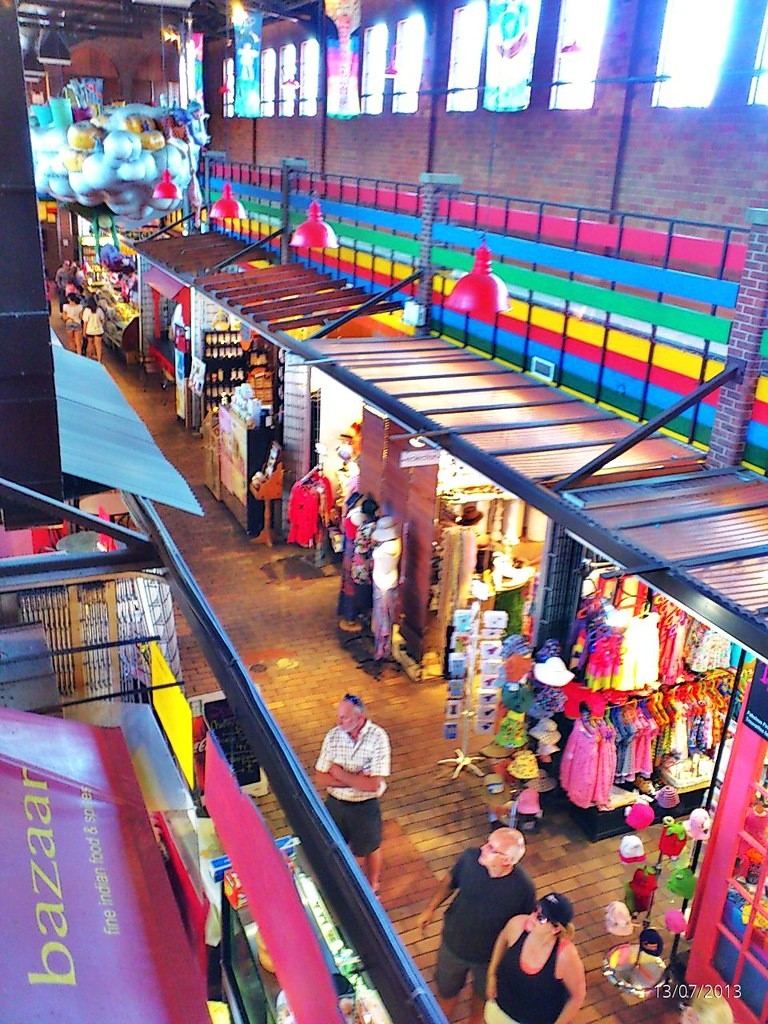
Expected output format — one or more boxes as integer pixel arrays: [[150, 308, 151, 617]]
[[622, 864, 657, 914], [659, 816, 688, 857], [533, 891, 574, 930], [603, 900, 634, 936], [664, 909, 686, 934], [683, 808, 711, 840], [616, 834, 646, 863], [639, 928, 664, 957], [654, 785, 680, 808], [343, 491, 364, 518], [370, 517, 401, 540], [624, 803, 655, 831], [312, 440, 357, 484], [479, 635, 576, 818], [456, 506, 484, 526], [665, 867, 698, 899]]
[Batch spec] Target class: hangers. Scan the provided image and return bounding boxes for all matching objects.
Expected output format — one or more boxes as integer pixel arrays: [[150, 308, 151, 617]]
[[576, 592, 603, 619], [583, 667, 749, 742], [297, 462, 326, 496], [634, 602, 652, 620]]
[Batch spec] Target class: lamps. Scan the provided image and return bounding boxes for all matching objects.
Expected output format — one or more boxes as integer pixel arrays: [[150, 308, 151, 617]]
[[207, 0, 249, 222], [151, 0, 183, 201], [439, 0, 514, 314], [287, 0, 341, 250]]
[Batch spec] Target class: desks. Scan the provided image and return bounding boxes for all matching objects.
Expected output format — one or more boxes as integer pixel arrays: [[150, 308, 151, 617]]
[[78, 493, 131, 530], [57, 531, 128, 552]]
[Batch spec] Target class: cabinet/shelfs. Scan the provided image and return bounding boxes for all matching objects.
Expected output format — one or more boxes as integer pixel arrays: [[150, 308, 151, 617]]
[[220, 875, 362, 1024]]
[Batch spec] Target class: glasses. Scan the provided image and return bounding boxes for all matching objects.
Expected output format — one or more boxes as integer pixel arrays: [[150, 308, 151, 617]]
[[484, 840, 512, 856], [343, 693, 359, 706], [532, 905, 549, 924]]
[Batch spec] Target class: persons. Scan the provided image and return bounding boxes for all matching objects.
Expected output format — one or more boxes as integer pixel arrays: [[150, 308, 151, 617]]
[[313, 690, 392, 901], [677, 988, 737, 1024], [52, 258, 107, 363], [334, 461, 402, 661], [420, 827, 585, 1024]]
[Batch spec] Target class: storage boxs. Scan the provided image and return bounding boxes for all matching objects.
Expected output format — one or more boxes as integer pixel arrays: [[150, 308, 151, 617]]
[[223, 850, 294, 911], [658, 754, 716, 795], [209, 835, 296, 885]]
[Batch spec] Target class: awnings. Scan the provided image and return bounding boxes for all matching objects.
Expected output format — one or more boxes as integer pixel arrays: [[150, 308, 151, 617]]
[[48, 341, 209, 523]]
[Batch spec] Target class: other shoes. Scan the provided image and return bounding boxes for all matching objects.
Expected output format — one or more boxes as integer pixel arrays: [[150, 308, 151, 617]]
[[370, 881, 381, 900]]
